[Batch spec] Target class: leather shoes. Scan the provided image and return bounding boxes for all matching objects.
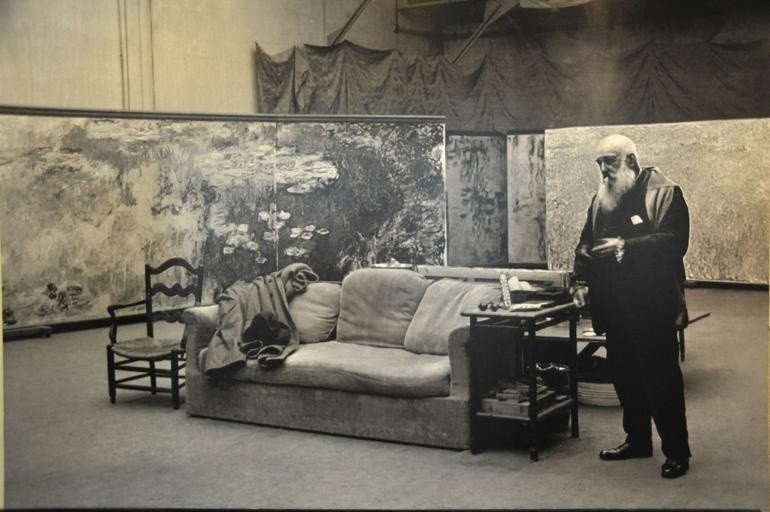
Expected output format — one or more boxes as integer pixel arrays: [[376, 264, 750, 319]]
[[661, 452, 694, 479], [599, 436, 654, 461]]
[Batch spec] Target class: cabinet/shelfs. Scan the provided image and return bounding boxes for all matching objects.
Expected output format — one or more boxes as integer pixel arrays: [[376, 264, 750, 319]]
[[463, 298, 581, 461]]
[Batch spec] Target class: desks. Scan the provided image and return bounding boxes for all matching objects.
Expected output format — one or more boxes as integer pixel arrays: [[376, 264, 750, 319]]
[[524, 310, 708, 407]]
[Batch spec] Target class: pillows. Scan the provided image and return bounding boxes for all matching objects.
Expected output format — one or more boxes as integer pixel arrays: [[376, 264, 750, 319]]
[[405, 279, 500, 356], [282, 281, 339, 342], [339, 269, 430, 347]]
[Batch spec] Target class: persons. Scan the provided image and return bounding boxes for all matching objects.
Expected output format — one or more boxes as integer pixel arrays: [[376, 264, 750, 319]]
[[570, 133, 692, 479]]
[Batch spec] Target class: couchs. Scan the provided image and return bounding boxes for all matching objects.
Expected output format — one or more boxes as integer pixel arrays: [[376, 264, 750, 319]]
[[183, 265, 504, 452], [414, 265, 591, 315]]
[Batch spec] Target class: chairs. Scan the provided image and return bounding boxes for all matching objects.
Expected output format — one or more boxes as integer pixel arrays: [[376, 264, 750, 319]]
[[107, 259, 203, 410]]
